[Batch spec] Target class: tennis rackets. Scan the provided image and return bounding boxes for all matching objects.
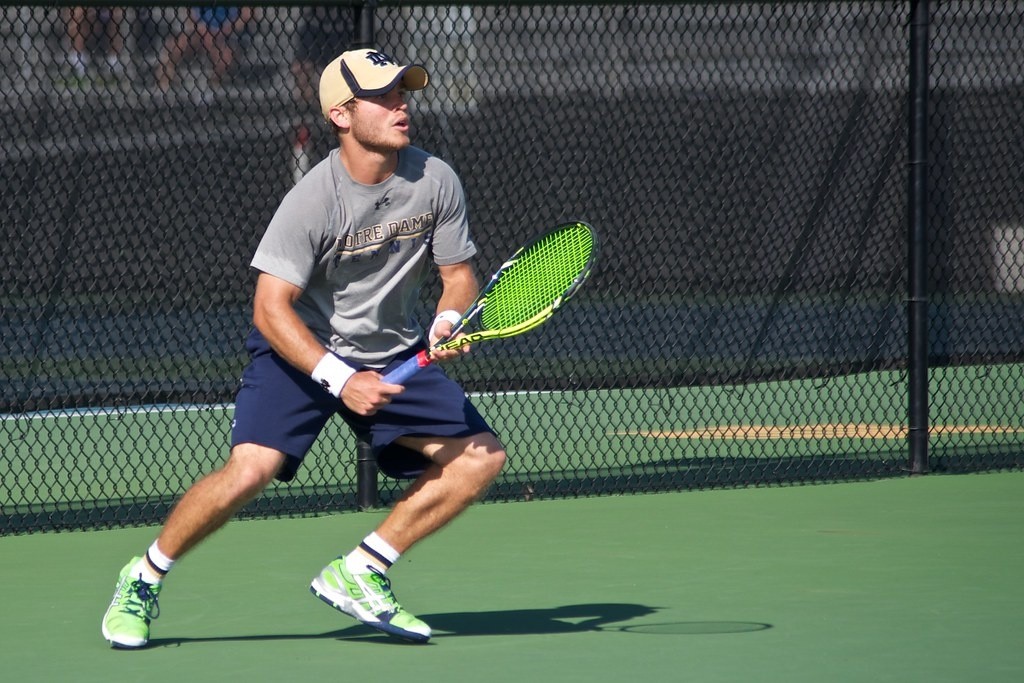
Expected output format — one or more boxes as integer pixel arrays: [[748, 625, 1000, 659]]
[[380, 221, 602, 382]]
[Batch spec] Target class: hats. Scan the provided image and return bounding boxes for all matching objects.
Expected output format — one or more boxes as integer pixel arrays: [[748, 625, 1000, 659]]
[[319, 49, 430, 121]]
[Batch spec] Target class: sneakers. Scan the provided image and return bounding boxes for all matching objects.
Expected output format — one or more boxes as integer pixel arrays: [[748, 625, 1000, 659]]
[[308, 554, 433, 644], [102, 556, 162, 649]]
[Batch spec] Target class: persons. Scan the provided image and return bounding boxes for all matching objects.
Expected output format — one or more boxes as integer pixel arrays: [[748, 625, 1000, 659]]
[[63, 2, 364, 132], [100, 47, 508, 652]]
